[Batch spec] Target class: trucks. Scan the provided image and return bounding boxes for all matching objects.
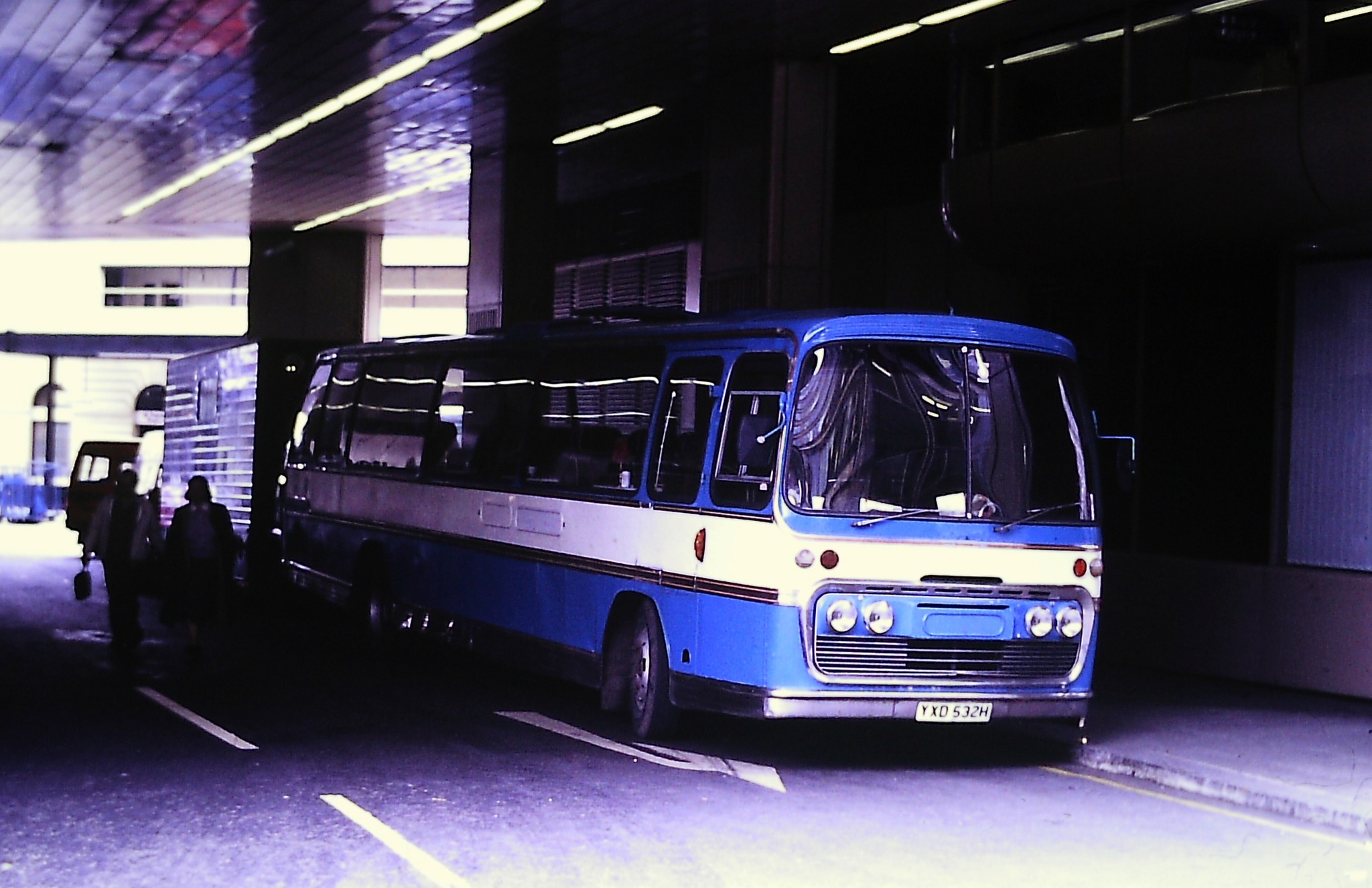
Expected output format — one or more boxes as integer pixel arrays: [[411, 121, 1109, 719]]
[[64, 334, 317, 612]]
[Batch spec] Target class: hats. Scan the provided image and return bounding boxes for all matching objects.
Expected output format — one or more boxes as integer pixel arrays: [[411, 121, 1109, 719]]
[[185, 476, 212, 501]]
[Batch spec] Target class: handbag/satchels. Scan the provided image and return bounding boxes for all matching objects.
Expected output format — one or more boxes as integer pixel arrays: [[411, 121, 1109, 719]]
[[74, 566, 91, 599]]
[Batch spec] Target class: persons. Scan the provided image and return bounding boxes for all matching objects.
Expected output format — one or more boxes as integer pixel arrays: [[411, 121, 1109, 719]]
[[80, 469, 167, 668], [164, 475, 238, 661]]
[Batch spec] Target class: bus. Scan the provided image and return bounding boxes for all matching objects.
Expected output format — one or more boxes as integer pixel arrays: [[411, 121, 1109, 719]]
[[266, 310, 1137, 752]]
